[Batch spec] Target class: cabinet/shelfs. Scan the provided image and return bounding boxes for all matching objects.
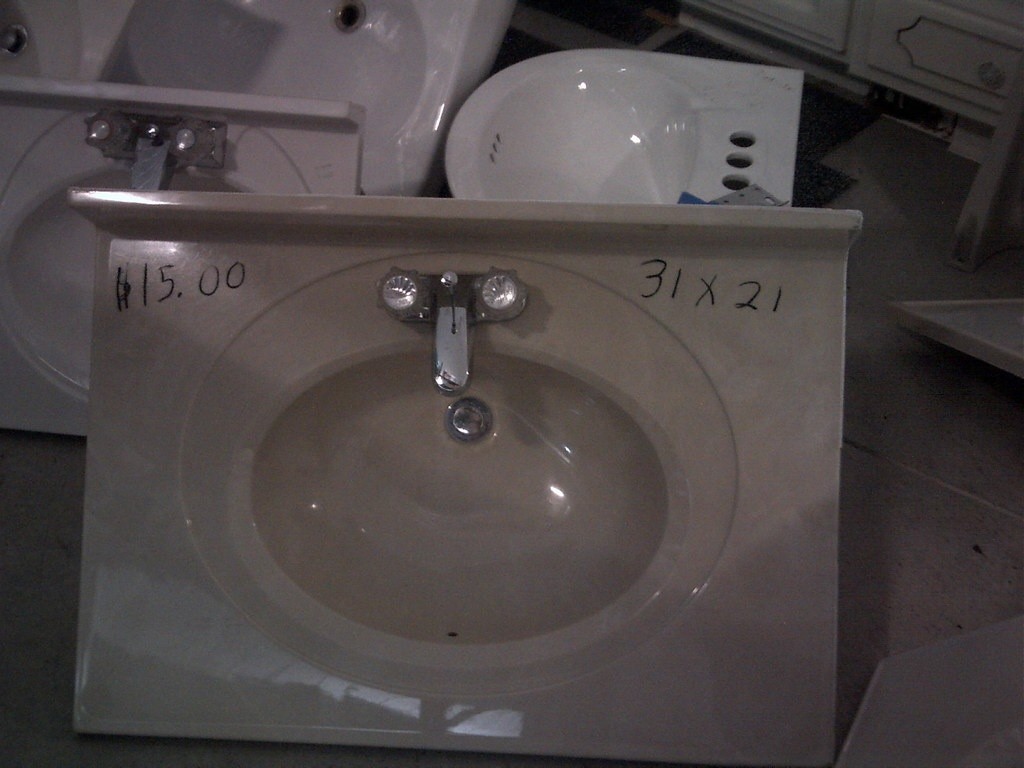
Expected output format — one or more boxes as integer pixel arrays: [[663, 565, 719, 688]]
[[678, 0, 1024, 271]]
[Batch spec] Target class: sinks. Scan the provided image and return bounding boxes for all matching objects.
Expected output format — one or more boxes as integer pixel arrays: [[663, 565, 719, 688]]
[[240, 351, 691, 652], [97, 0, 430, 159], [475, 59, 701, 203], [7, 170, 255, 387]]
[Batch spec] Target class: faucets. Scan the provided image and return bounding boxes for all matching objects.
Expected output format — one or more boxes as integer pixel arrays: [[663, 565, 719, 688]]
[[432, 272, 476, 397], [128, 125, 172, 189]]
